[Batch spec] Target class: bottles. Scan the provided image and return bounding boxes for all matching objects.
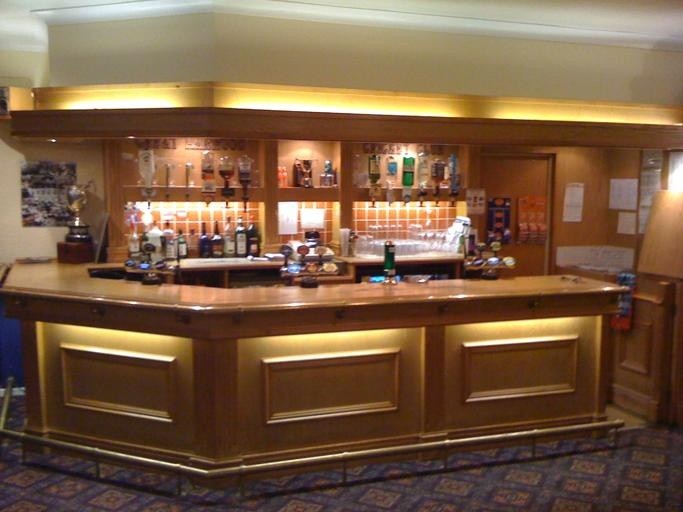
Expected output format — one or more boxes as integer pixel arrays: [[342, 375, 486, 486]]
[[124, 214, 260, 258], [364, 150, 461, 201], [137, 147, 254, 201], [304, 232, 320, 248], [276, 166, 289, 189], [383, 240, 396, 284], [459, 224, 476, 256]]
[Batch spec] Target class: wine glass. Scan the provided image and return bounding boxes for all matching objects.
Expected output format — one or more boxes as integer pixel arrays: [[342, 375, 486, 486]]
[[417, 232, 447, 253]]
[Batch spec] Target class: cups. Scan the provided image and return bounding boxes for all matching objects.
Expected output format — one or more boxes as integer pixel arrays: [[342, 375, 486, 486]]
[[355, 223, 422, 256], [339, 227, 350, 255]]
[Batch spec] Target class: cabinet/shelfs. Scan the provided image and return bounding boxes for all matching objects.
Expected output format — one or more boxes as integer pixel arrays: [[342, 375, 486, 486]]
[[347, 143, 481, 256], [271, 140, 347, 256], [167, 265, 347, 291], [104, 137, 271, 258], [351, 261, 461, 284]]
